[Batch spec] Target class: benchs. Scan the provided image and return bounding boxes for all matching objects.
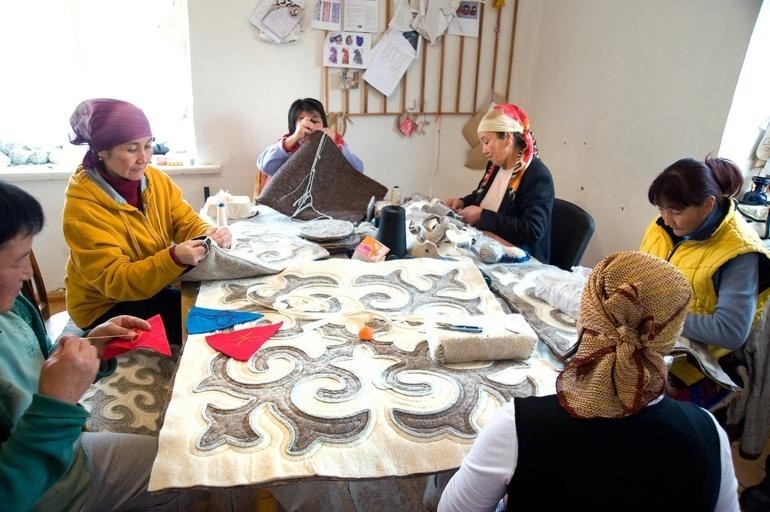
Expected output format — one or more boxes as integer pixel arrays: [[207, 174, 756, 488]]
[[549, 197, 596, 272]]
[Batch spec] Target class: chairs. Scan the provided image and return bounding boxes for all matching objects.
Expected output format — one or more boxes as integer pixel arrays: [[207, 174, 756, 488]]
[[22, 249, 70, 345]]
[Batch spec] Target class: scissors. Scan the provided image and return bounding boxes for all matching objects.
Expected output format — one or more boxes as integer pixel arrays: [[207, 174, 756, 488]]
[[433, 320, 484, 334]]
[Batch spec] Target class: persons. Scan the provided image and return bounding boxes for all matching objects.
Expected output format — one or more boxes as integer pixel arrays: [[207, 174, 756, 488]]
[[61, 96, 233, 345], [255, 97, 364, 178], [436, 250, 742, 512], [442, 102, 556, 264], [639, 155, 770, 413], [0, 180, 189, 512]]
